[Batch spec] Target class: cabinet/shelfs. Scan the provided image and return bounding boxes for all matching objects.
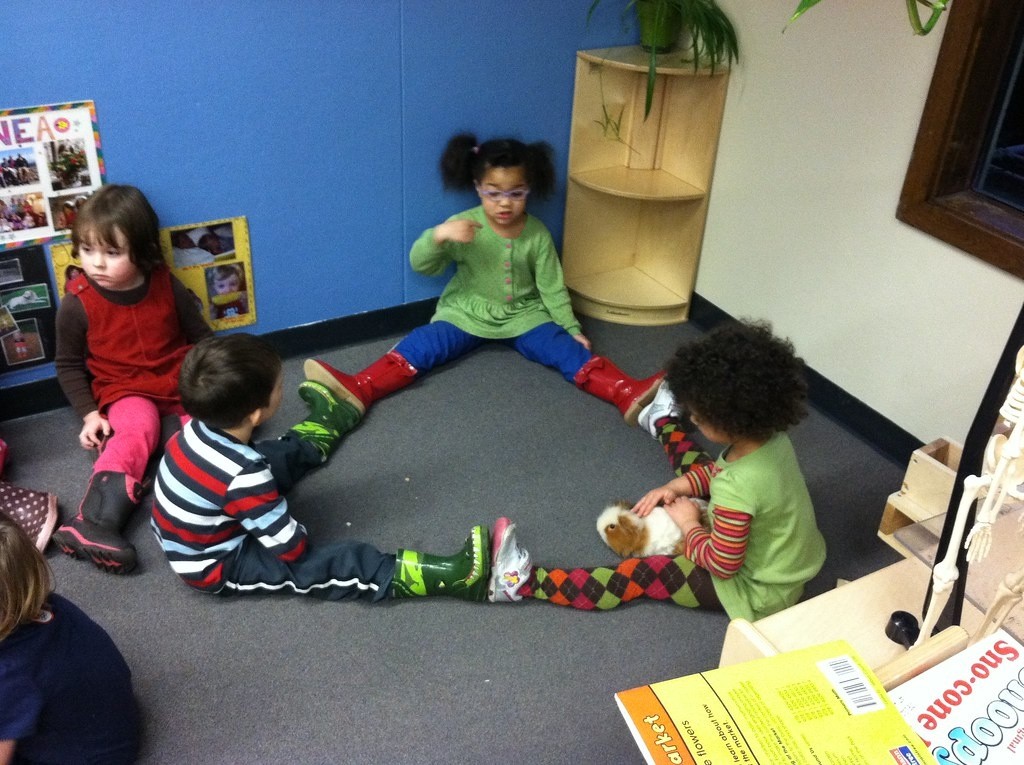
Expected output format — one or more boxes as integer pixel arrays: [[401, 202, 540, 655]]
[[563, 46, 730, 329]]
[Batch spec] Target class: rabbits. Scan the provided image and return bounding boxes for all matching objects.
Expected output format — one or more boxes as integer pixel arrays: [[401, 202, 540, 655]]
[[594, 497, 710, 559]]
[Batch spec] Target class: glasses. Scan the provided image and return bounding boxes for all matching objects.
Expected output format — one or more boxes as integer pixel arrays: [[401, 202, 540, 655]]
[[474, 180, 529, 201]]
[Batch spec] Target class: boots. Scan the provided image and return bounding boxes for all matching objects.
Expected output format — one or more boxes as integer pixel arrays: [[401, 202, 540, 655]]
[[95, 414, 183, 470], [574, 354, 668, 428], [53, 471, 146, 575], [390, 525, 489, 604], [304, 349, 418, 415], [289, 380, 363, 462]]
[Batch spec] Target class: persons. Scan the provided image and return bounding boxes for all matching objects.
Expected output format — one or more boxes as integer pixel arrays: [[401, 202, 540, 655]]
[[50, 184, 215, 577], [0, 140, 93, 231], [150, 334, 488, 612], [489, 323, 825, 623], [13, 330, 30, 360], [209, 265, 251, 318], [302, 131, 670, 429], [1, 510, 139, 765]]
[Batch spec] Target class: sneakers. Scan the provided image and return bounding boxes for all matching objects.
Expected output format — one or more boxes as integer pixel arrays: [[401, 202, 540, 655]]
[[638, 381, 681, 439], [489, 517, 531, 602]]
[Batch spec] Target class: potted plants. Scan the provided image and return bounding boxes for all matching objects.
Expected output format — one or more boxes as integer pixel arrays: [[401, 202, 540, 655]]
[[592, 0, 952, 147]]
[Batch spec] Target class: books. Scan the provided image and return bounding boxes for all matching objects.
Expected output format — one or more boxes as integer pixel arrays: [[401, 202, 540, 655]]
[[614, 626, 1024, 765]]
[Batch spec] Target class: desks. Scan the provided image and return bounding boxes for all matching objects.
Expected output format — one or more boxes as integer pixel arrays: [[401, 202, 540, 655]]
[[718, 505, 1024, 669]]
